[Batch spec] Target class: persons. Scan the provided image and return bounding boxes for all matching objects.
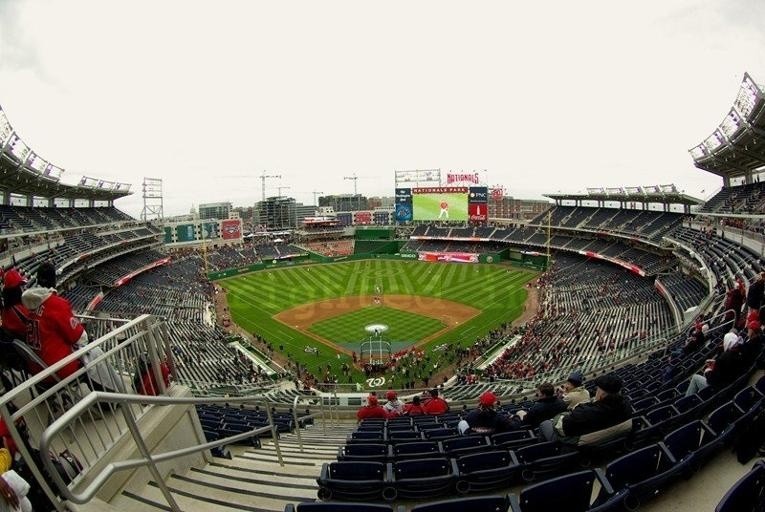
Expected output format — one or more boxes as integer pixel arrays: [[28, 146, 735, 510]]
[[1, 174, 765, 512]]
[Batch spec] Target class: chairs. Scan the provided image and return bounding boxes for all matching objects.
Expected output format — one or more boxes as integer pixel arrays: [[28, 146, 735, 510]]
[[0, 181, 765, 512]]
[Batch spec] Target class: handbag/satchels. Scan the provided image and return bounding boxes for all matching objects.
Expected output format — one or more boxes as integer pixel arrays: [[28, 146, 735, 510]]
[[132, 352, 170, 406]]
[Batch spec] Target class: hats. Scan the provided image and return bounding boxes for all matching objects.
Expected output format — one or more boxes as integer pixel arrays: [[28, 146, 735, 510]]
[[367, 395, 378, 404], [385, 391, 397, 400], [594, 375, 621, 393], [481, 392, 497, 406], [567, 373, 583, 387], [4, 271, 27, 287], [537, 384, 554, 395]]
